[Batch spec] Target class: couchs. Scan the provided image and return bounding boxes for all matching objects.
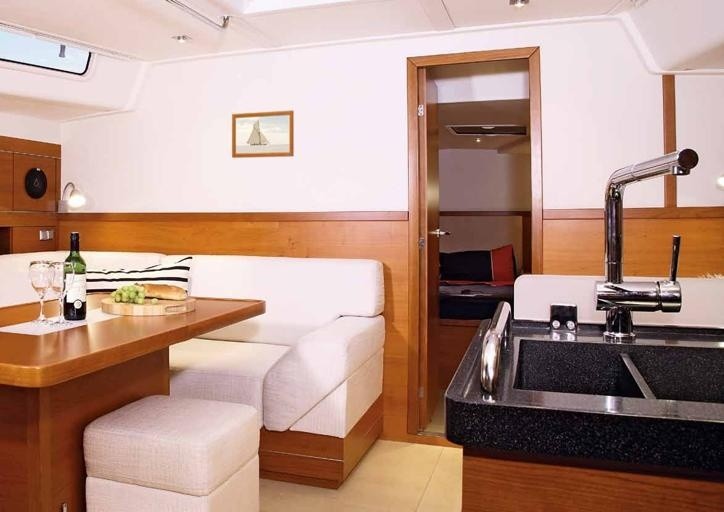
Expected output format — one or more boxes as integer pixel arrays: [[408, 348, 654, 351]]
[[0, 250, 385, 490]]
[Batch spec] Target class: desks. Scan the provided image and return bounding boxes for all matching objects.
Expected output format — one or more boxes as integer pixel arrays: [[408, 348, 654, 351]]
[[0, 289, 265, 512]]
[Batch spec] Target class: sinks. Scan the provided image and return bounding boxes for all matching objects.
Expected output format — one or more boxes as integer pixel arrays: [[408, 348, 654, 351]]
[[513, 339, 724, 404]]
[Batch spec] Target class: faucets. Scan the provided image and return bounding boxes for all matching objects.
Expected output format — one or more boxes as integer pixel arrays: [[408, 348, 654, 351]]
[[593, 149, 699, 342]]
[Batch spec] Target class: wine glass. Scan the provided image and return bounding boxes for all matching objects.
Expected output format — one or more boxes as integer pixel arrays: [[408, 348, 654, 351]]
[[50, 262, 73, 328], [28, 261, 53, 328]]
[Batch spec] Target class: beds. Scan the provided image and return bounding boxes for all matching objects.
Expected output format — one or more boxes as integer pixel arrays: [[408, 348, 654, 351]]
[[439, 216, 523, 327]]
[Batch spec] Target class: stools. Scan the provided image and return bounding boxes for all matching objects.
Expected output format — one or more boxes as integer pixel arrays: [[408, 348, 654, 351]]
[[84, 394, 259, 512]]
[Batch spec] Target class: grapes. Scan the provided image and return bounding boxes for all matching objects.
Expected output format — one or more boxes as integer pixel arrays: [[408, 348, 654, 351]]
[[110, 285, 147, 306]]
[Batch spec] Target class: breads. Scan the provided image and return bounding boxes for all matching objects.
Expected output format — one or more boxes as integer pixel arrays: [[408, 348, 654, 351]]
[[140, 283, 189, 301]]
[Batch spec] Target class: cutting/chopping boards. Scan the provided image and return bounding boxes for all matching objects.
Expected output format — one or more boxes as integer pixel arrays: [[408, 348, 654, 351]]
[[101, 295, 194, 313]]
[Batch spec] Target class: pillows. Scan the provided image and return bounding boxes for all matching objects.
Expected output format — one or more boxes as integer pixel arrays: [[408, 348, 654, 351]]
[[85, 257, 192, 294]]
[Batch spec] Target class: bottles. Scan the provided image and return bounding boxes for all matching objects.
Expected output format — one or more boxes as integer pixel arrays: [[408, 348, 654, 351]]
[[63, 232, 86, 321]]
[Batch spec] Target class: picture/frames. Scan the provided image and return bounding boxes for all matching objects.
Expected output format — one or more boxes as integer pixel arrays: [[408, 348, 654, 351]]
[[232, 111, 294, 157]]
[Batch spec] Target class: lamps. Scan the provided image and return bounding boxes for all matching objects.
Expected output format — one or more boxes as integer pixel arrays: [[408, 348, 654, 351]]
[[58, 182, 88, 213]]
